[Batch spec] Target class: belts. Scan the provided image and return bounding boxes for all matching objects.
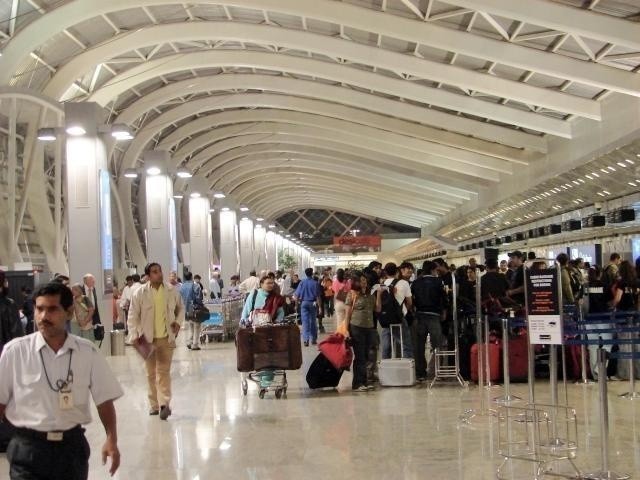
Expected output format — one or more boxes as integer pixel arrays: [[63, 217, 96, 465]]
[[14, 427, 87, 441]]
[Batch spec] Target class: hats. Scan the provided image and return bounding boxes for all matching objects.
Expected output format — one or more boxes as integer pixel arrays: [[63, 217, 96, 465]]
[[507, 251, 523, 258]]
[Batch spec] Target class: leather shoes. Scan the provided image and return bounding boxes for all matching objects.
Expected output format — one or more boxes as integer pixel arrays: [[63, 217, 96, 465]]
[[149, 410, 158, 416], [160, 408, 172, 420]]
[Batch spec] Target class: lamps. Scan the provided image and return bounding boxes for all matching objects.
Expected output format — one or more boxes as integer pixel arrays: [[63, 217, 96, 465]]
[[214, 189, 226, 199], [178, 168, 192, 178], [36, 128, 57, 140], [173, 192, 184, 199], [111, 124, 130, 137], [65, 120, 86, 135], [117, 132, 134, 140], [124, 168, 138, 178]]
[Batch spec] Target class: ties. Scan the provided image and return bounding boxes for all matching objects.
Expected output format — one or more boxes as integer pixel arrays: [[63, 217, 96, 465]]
[[87, 288, 92, 301]]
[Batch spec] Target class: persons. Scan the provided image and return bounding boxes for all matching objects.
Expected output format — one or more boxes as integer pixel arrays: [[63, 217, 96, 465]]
[[81, 273, 102, 325], [127, 262, 186, 420], [1, 268, 24, 453], [267, 272, 280, 295], [0, 282, 124, 480], [50, 272, 72, 335], [209, 272, 221, 299], [225, 276, 240, 296], [374, 262, 413, 359], [240, 270, 260, 302], [119, 275, 133, 334], [72, 283, 96, 343], [128, 274, 142, 301], [291, 274, 302, 325], [343, 271, 384, 391], [275, 270, 285, 296], [21, 284, 35, 334], [321, 276, 334, 318], [399, 262, 413, 281], [180, 271, 203, 350], [194, 274, 203, 290], [332, 268, 351, 333], [323, 266, 335, 311], [368, 261, 382, 276], [217, 274, 224, 289], [239, 276, 284, 393], [432, 257, 453, 292], [410, 260, 447, 380], [455, 251, 640, 382], [293, 268, 317, 347], [312, 276, 325, 329], [165, 270, 183, 290]]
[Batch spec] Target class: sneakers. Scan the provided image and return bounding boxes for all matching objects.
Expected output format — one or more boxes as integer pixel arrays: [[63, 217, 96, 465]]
[[352, 384, 375, 392], [186, 343, 200, 351], [303, 341, 310, 346], [312, 340, 318, 345]]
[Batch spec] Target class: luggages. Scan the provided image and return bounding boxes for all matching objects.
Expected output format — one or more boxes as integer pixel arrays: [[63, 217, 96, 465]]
[[377, 357, 416, 387], [502, 334, 531, 381], [305, 352, 343, 388], [231, 321, 304, 371], [469, 343, 502, 382]]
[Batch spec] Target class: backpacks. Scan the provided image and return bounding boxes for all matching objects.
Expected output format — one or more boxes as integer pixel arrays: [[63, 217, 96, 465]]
[[372, 278, 406, 329]]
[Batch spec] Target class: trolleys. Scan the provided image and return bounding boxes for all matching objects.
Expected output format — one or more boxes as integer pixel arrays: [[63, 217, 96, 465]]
[[240, 316, 293, 399], [283, 298, 301, 325], [200, 293, 246, 343]]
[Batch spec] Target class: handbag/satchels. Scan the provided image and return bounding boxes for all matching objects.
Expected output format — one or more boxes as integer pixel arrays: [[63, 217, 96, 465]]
[[81, 295, 104, 342], [188, 281, 210, 321], [336, 280, 350, 303], [336, 290, 358, 338]]
